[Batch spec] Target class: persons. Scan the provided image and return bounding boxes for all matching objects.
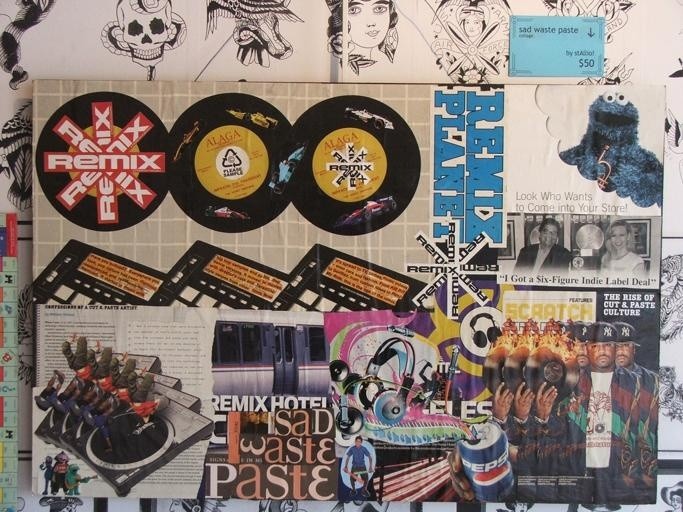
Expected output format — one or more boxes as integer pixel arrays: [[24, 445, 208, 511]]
[[459, 6, 487, 43], [514, 218, 571, 276], [326, 0, 398, 75], [447, 318, 658, 504], [599, 221, 645, 280], [342, 435, 376, 499], [33, 335, 163, 453], [241, 411, 268, 433]]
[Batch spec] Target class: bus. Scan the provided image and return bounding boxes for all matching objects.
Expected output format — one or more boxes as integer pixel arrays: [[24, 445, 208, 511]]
[[213, 320, 331, 396]]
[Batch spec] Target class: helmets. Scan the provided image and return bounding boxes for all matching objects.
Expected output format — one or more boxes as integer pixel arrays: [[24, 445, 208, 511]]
[[572, 321, 586, 343], [585, 321, 616, 343], [615, 322, 639, 344]]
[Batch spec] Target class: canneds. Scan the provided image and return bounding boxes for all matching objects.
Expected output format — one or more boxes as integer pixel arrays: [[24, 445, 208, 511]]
[[458, 420, 514, 503]]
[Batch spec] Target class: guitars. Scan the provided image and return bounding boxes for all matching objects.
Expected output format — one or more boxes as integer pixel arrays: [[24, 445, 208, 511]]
[[64, 475, 99, 490]]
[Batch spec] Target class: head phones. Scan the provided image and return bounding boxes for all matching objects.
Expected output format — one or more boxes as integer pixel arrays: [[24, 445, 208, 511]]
[[329, 337, 415, 434]]
